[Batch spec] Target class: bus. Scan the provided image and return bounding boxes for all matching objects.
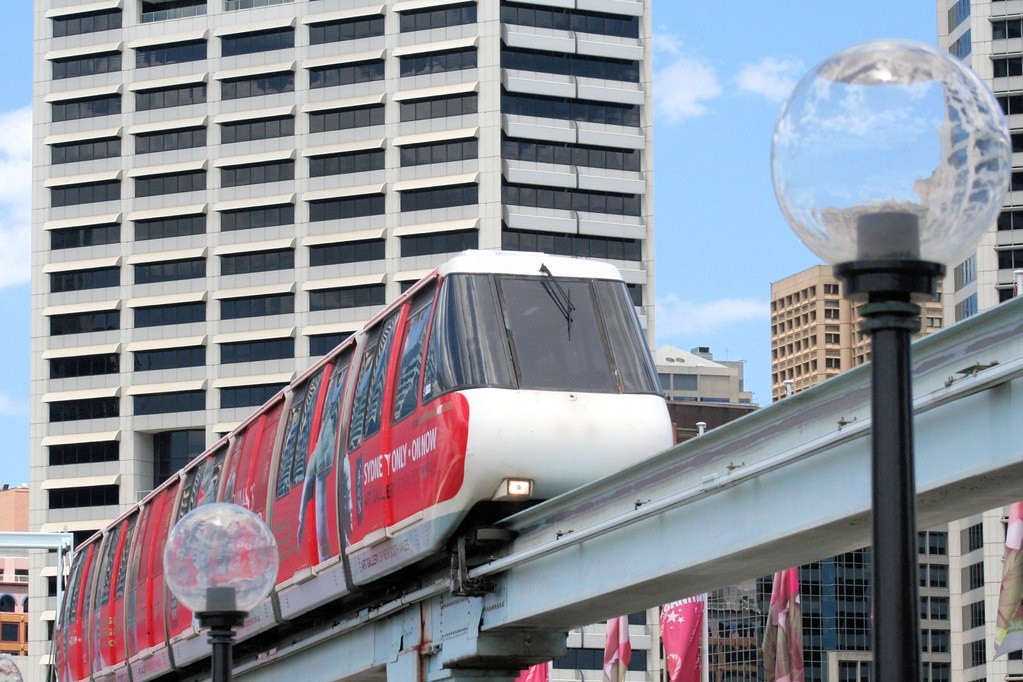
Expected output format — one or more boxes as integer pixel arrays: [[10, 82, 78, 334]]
[[48, 248, 677, 682]]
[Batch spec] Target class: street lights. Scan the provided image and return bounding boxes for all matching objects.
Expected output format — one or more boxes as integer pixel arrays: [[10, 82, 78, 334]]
[[162, 500, 280, 682], [769, 36, 1013, 682], [57, 541, 71, 624]]
